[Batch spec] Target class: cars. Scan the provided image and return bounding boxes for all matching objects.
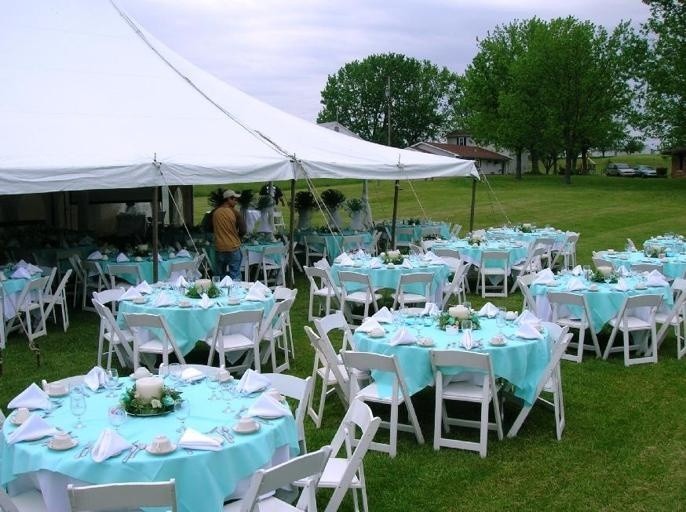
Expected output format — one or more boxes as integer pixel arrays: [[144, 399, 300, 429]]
[[605, 163, 636, 177], [633, 163, 657, 177]]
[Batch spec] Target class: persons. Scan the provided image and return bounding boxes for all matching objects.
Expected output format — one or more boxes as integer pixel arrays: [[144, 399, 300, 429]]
[[259, 184, 287, 208], [207, 189, 247, 282]]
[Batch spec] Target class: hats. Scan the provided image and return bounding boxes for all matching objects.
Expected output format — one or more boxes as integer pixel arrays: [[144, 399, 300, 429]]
[[222, 189, 242, 199]]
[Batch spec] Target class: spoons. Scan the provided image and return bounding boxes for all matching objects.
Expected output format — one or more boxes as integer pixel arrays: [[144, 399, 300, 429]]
[[223, 426, 233, 438], [82, 441, 94, 456], [131, 442, 146, 457]]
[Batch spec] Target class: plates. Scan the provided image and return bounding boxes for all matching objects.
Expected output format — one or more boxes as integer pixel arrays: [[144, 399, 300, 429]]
[[146, 445, 176, 453], [46, 438, 77, 449], [258, 416, 280, 420], [11, 417, 28, 425], [232, 423, 259, 433], [44, 388, 64, 396]]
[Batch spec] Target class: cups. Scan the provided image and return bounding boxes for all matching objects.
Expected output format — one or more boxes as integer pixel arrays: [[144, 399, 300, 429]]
[[228, 298, 240, 303], [420, 336, 433, 344], [492, 336, 504, 343], [151, 436, 171, 450], [134, 296, 144, 302], [180, 298, 192, 305]]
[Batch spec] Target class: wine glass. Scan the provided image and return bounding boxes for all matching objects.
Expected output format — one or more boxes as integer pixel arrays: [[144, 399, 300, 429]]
[[408, 250, 415, 262], [212, 276, 219, 290], [70, 394, 88, 427], [108, 407, 127, 433], [604, 272, 611, 288], [104, 369, 118, 397], [174, 288, 184, 304], [231, 285, 239, 299], [496, 305, 506, 339], [68, 381, 85, 396], [461, 319, 472, 338], [205, 369, 235, 412], [174, 399, 190, 432], [159, 365, 183, 389], [463, 302, 471, 316], [392, 300, 408, 332], [415, 317, 424, 337]]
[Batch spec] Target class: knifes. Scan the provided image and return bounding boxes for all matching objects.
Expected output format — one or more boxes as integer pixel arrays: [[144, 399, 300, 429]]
[[122, 444, 138, 462]]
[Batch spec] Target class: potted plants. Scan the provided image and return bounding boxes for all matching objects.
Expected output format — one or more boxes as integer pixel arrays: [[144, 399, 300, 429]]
[[318, 189, 346, 227], [343, 197, 364, 230], [288, 191, 323, 231]]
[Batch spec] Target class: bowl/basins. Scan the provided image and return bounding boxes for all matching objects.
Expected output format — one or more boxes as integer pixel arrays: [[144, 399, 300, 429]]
[[51, 433, 70, 447], [237, 416, 255, 430], [14, 407, 27, 422], [49, 382, 62, 392]]
